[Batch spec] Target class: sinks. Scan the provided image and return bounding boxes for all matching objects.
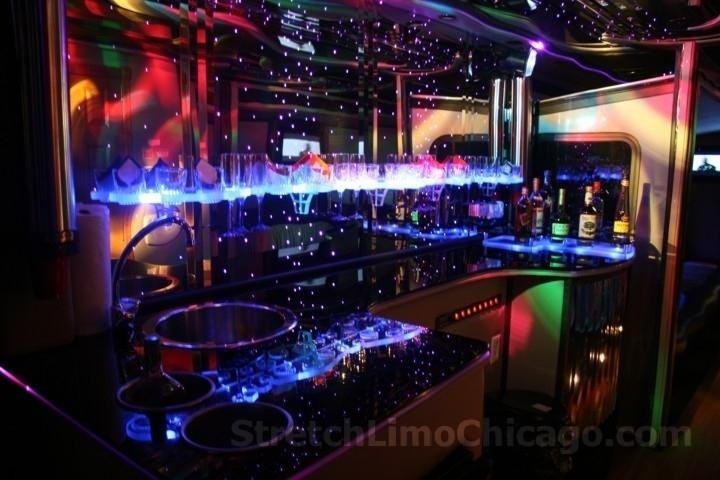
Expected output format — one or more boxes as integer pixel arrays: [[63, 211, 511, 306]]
[[141, 295, 301, 371]]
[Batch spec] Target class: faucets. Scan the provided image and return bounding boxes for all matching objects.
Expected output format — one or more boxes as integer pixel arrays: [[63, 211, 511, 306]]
[[113, 215, 196, 321]]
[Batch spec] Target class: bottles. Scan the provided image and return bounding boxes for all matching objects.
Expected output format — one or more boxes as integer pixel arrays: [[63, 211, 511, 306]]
[[578, 185, 599, 241], [514, 187, 533, 243], [593, 181, 604, 232], [550, 188, 571, 240], [528, 177, 545, 241], [612, 179, 634, 245]]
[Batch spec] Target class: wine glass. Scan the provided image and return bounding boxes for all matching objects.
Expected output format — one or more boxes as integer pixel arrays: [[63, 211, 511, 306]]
[[101, 155, 504, 241]]
